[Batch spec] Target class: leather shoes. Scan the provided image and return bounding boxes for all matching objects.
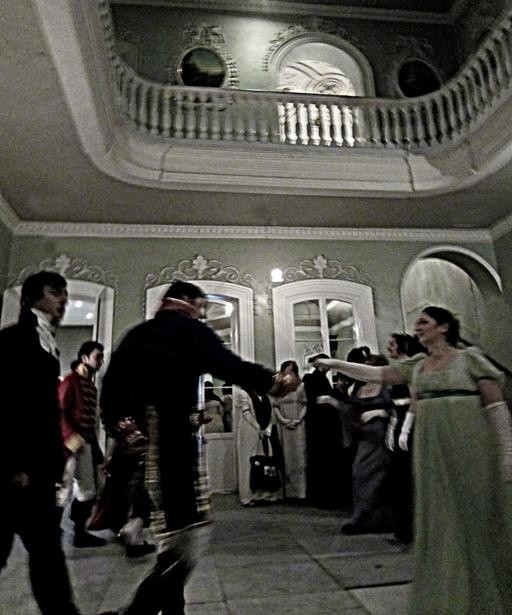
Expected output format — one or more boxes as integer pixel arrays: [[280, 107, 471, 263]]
[[126, 540, 157, 557], [73, 533, 108, 548]]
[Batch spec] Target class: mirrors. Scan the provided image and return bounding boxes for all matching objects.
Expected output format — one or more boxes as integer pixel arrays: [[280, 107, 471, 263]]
[[168, 24, 239, 110], [386, 36, 449, 99]]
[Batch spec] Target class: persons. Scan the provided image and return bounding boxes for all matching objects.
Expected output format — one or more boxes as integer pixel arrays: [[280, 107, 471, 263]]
[[101, 279, 308, 615], [55, 339, 109, 546], [303, 306, 511, 615], [0, 270, 82, 615]]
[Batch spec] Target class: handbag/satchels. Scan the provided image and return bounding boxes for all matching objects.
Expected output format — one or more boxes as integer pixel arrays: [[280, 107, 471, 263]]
[[249, 435, 283, 494]]
